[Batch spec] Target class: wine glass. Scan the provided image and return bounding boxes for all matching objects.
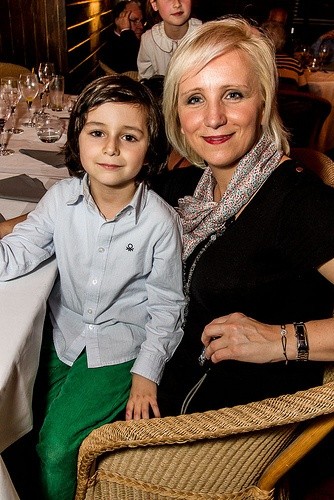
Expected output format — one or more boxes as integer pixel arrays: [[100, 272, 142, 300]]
[[0, 92, 15, 157], [38, 62, 55, 107], [18, 73, 39, 127], [0, 77, 24, 134]]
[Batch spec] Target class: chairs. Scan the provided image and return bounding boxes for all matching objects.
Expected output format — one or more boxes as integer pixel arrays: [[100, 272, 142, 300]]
[[76, 362, 334, 500], [276, 89, 334, 153]]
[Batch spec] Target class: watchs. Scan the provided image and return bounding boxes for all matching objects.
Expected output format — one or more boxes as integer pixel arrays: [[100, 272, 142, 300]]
[[292, 322, 309, 361]]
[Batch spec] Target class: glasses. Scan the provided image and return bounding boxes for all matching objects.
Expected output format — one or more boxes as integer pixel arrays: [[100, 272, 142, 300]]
[[130, 19, 148, 25]]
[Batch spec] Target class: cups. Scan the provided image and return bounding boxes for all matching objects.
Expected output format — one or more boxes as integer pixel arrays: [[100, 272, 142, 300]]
[[49, 75, 64, 111], [35, 115, 65, 144]]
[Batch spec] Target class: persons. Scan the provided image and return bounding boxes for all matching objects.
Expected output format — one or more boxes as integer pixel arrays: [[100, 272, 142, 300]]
[[0, 74, 188, 500], [99, 0, 144, 72], [259, 9, 333, 94], [138, 0, 204, 78], [148, 19, 333, 500]]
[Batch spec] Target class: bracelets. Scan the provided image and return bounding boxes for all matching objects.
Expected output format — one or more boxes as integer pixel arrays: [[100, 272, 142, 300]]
[[281, 324, 288, 364]]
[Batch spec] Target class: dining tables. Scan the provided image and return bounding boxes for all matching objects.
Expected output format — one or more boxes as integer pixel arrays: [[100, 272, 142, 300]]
[[305, 70, 334, 95], [0, 91, 103, 500]]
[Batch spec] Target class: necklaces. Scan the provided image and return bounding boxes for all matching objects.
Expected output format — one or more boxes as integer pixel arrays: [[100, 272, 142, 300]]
[[182, 224, 225, 329]]
